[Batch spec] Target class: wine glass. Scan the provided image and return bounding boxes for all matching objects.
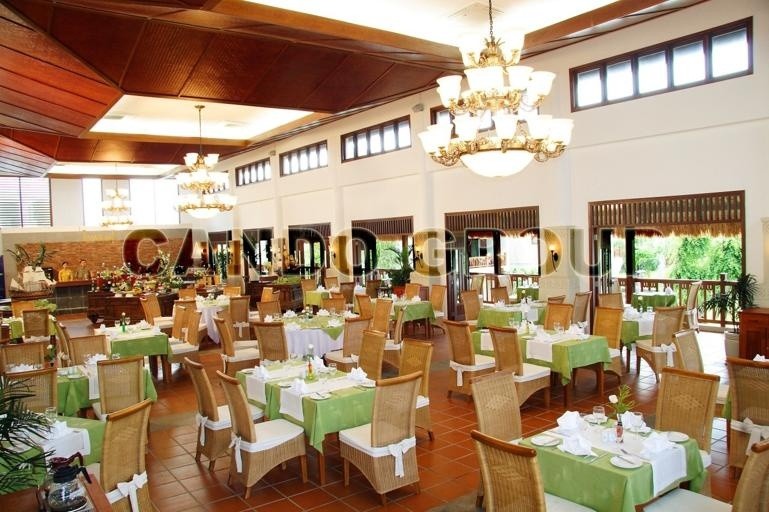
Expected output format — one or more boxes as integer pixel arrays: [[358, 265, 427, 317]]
[[270, 352, 340, 396], [508, 316, 566, 342], [83, 353, 91, 368], [630, 411, 645, 441], [592, 406, 606, 429], [43, 404, 57, 434], [114, 317, 131, 336]]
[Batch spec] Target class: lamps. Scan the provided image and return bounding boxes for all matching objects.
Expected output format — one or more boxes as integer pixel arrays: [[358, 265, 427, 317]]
[[175, 103, 238, 221], [417, 0, 575, 179], [100, 179, 133, 231]]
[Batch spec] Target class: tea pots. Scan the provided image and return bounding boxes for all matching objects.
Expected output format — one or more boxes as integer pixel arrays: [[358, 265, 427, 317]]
[[43, 451, 91, 510]]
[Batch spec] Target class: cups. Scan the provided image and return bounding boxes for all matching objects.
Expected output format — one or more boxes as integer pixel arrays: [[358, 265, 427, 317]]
[[111, 353, 121, 359]]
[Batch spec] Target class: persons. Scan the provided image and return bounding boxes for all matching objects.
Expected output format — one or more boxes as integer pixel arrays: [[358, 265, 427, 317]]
[[200, 248, 208, 266], [75, 259, 92, 281], [57, 261, 73, 282]]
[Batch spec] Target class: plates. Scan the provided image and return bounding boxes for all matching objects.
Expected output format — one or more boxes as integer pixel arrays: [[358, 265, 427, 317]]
[[532, 432, 558, 449], [662, 429, 690, 444], [585, 413, 608, 424], [612, 453, 642, 471], [237, 362, 377, 401]]
